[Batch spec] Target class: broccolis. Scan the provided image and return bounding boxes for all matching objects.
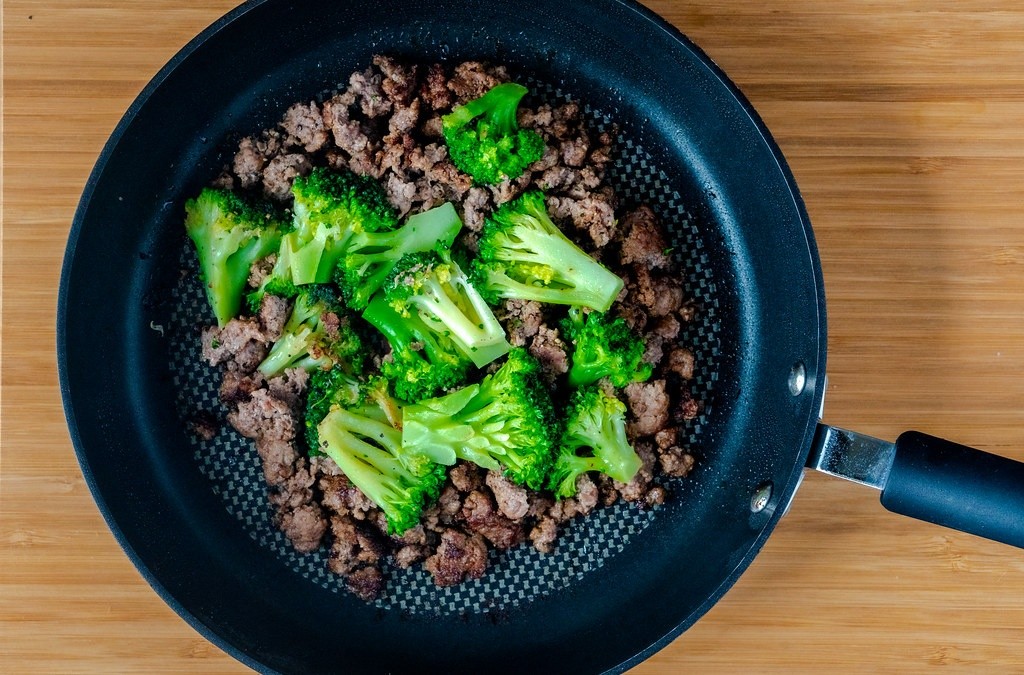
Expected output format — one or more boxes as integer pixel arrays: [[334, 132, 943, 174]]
[[182, 81, 653, 535]]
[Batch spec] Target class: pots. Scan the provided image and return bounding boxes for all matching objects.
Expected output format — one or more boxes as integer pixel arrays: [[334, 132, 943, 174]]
[[54, 2, 1021, 671]]
